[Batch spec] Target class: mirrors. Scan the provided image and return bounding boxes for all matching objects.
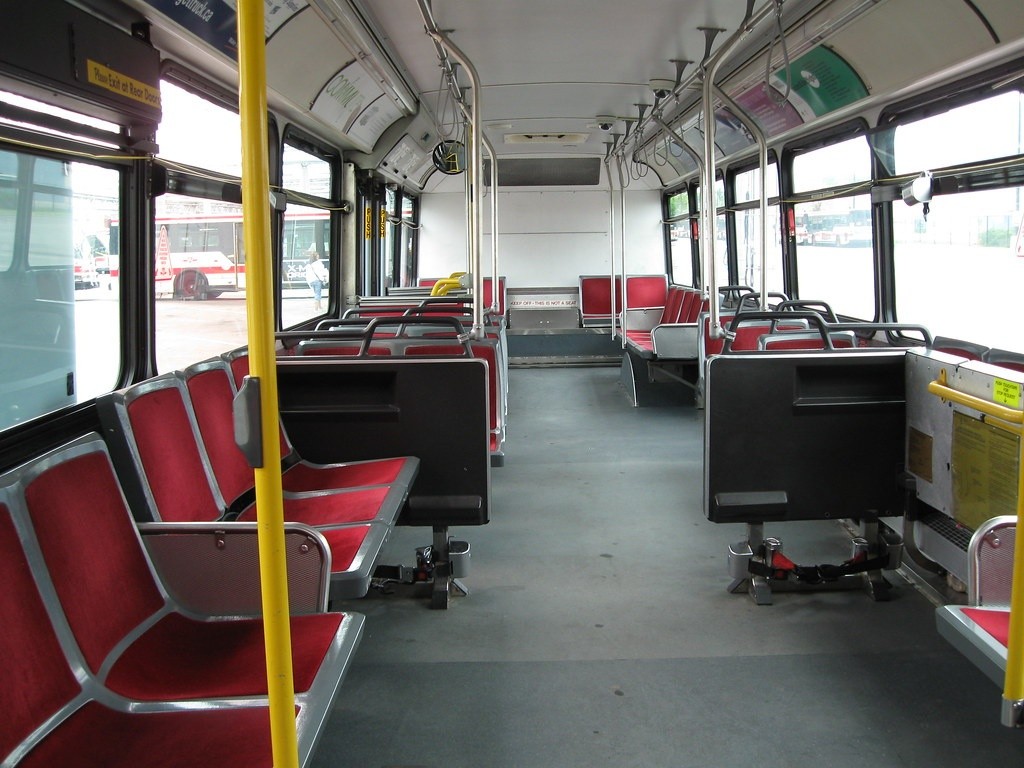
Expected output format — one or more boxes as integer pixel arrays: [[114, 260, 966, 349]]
[[433, 140, 465, 175]]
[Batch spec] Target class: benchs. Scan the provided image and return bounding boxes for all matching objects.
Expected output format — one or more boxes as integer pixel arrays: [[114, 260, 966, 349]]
[[579, 274, 1024, 730], [0, 272, 506, 768]]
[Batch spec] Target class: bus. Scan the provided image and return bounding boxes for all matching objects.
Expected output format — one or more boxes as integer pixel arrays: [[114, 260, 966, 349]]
[[105, 210, 414, 301], [796, 209, 871, 246], [0, 149, 78, 428]]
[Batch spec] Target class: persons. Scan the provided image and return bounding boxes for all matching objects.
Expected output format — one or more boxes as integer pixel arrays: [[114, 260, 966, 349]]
[[306, 250, 327, 313]]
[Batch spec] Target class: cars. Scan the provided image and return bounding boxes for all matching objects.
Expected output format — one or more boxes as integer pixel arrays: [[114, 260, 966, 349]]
[[75, 235, 107, 286]]
